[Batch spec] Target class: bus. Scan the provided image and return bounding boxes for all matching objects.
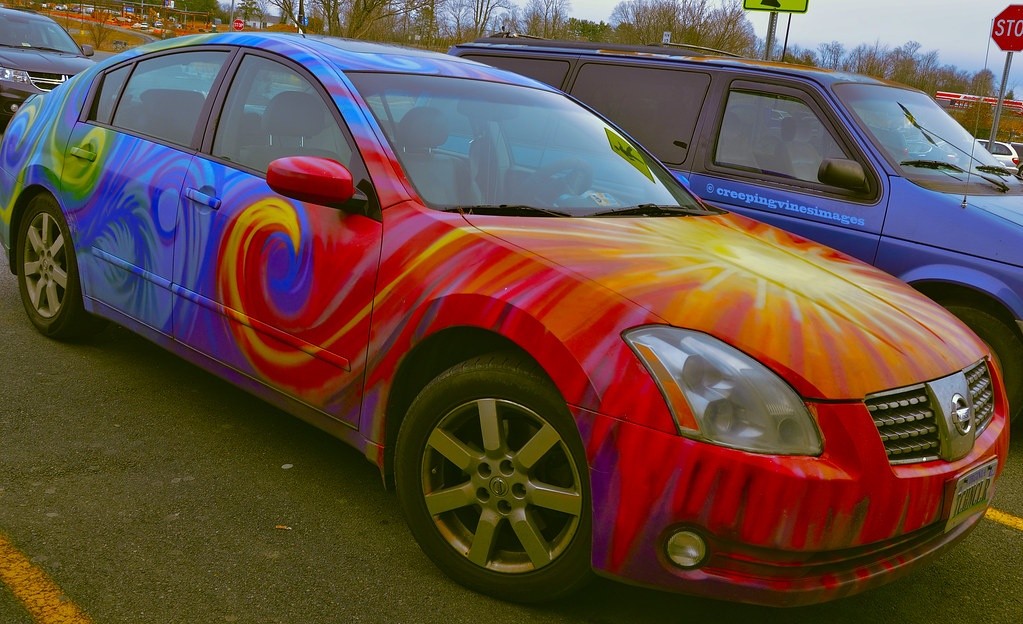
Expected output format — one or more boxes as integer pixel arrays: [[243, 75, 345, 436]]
[[934, 90, 1023, 116]]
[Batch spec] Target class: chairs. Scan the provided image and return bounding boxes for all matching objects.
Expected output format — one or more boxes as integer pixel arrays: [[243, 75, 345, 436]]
[[394, 107, 482, 205], [240, 91, 342, 169], [716, 111, 762, 174], [776, 116, 821, 182]]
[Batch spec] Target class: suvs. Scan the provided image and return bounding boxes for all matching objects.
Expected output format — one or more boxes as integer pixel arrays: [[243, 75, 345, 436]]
[[444, 34, 1020, 440]]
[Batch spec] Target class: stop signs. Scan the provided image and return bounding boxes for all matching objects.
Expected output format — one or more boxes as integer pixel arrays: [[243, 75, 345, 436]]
[[233, 19, 245, 32], [991, 4, 1023, 52]]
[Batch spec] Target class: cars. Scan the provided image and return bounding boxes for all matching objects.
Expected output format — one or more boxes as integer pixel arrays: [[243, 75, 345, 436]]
[[73, 5, 82, 13], [974, 139, 1020, 175], [1010, 142, 1023, 180], [141, 23, 149, 30], [154, 21, 164, 28], [0, 33, 1011, 608], [55, 5, 68, 11], [0, 9, 102, 131], [86, 7, 95, 14]]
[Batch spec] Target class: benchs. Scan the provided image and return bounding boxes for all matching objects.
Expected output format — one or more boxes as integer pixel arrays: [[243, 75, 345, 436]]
[[136, 88, 259, 138]]
